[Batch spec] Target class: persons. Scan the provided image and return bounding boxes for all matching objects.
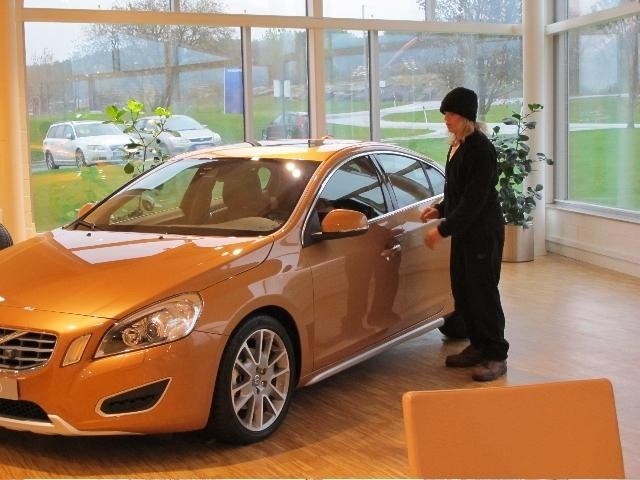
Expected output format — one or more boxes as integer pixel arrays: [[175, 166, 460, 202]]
[[422, 87, 509, 380]]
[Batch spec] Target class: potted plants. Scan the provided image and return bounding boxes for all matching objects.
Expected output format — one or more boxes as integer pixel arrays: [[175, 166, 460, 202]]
[[484, 99, 556, 263]]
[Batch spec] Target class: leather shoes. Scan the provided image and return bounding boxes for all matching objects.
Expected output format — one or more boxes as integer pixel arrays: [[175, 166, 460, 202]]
[[471, 361, 508, 381], [445, 344, 489, 367]]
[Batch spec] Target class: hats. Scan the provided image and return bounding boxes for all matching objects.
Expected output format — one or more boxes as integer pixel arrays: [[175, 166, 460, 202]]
[[439, 86, 478, 122]]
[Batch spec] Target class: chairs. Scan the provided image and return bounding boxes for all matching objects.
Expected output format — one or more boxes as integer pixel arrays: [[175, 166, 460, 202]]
[[210, 168, 266, 222], [396, 373, 628, 478]]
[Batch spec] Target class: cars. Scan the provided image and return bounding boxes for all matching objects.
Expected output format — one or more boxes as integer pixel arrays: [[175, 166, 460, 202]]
[[41, 117, 145, 171], [126, 113, 224, 160], [0, 134, 453, 457], [258, 109, 309, 141]]
